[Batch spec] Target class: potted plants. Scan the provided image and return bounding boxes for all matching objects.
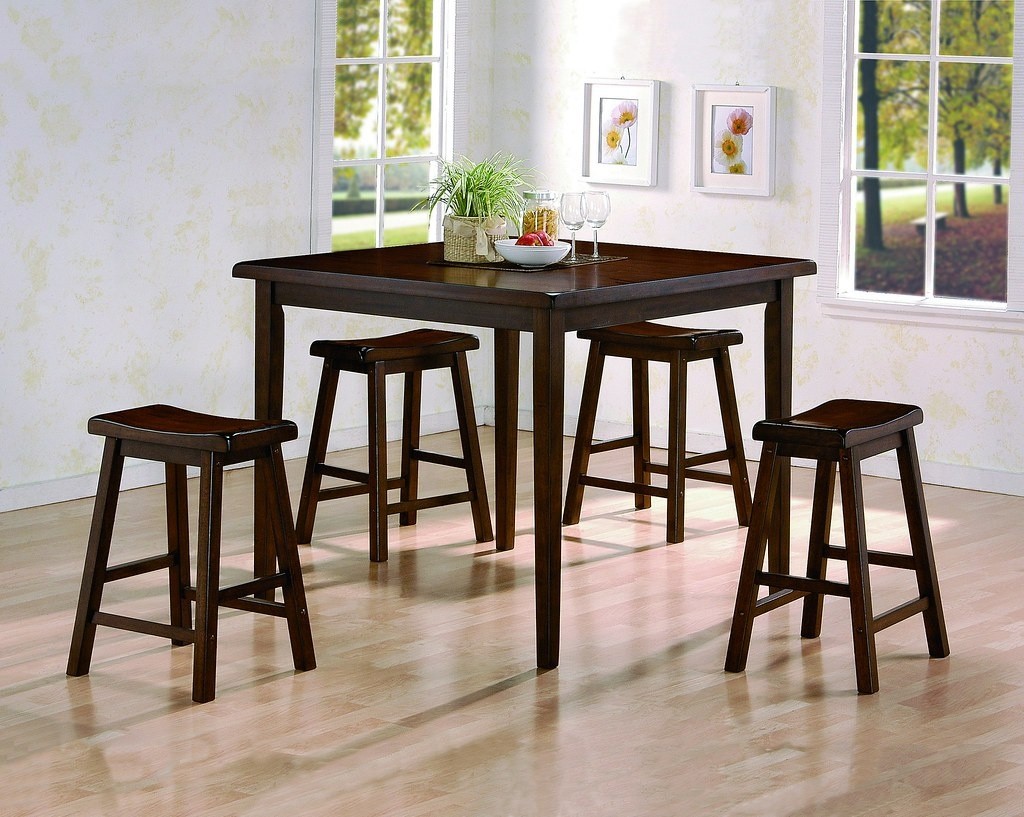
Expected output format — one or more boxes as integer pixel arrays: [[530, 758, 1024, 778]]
[[408, 150, 547, 264]]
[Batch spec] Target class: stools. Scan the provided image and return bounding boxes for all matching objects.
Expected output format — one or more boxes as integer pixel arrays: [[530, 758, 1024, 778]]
[[562, 322, 752, 544], [296, 327, 493, 562], [723, 399, 951, 695], [65, 403, 316, 706]]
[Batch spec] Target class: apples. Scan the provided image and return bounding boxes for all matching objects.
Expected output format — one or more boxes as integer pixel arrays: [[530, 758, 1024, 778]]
[[515, 230, 554, 246]]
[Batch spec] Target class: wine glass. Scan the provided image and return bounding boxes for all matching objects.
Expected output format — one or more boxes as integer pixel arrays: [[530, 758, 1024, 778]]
[[580, 191, 611, 261], [559, 192, 589, 264]]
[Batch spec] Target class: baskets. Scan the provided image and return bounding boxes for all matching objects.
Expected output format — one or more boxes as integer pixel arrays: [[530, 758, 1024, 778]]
[[442, 214, 508, 264]]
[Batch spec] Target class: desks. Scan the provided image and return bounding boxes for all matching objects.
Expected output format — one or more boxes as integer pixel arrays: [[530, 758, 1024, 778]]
[[233, 238, 816, 677]]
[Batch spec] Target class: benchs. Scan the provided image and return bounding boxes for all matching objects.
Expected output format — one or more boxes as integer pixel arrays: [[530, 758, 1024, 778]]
[[911, 212, 949, 236]]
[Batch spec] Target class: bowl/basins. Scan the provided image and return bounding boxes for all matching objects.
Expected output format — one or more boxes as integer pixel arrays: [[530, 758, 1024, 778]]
[[493, 238, 572, 268]]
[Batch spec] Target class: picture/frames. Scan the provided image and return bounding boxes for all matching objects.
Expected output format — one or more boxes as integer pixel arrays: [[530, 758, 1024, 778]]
[[689, 84, 776, 198], [581, 77, 661, 188]]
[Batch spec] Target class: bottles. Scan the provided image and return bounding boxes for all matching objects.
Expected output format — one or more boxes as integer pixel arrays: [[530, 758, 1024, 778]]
[[519, 189, 559, 242]]
